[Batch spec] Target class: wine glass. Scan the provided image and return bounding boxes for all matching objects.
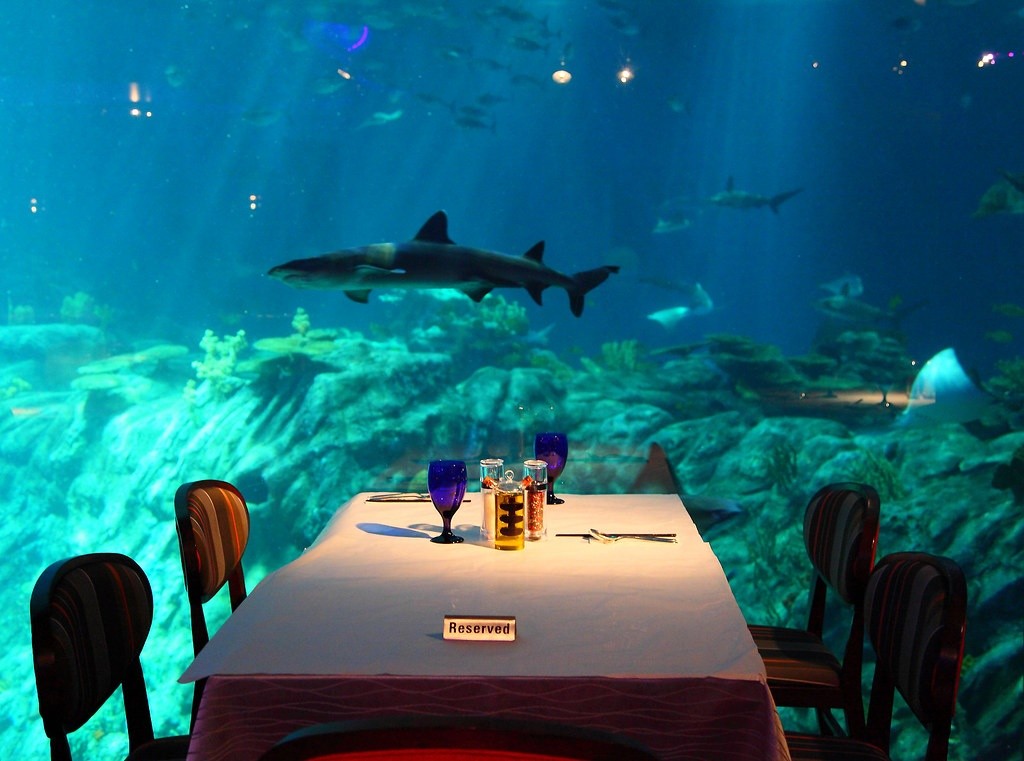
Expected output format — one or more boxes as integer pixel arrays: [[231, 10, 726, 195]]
[[535, 431, 568, 504], [428, 460, 466, 544]]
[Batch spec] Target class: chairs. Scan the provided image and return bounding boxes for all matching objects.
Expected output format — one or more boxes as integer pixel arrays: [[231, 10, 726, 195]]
[[740, 479, 884, 729], [173, 480, 249, 651], [27, 551, 196, 761], [783, 550, 965, 761]]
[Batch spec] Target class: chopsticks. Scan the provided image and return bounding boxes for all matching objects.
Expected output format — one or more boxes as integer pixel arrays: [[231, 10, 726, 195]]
[[555, 533, 677, 536], [366, 500, 472, 503]]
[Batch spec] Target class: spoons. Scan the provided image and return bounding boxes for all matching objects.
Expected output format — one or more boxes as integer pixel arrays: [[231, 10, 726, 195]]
[[589, 530, 676, 542], [369, 492, 431, 499]]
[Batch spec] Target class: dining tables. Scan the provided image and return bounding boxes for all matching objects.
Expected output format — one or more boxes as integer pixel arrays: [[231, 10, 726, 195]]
[[172, 491, 792, 759]]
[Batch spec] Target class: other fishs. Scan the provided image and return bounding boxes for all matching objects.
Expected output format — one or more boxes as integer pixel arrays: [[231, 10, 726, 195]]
[[623, 442, 750, 542], [635, 213, 1024, 418], [160, 1, 651, 132]]
